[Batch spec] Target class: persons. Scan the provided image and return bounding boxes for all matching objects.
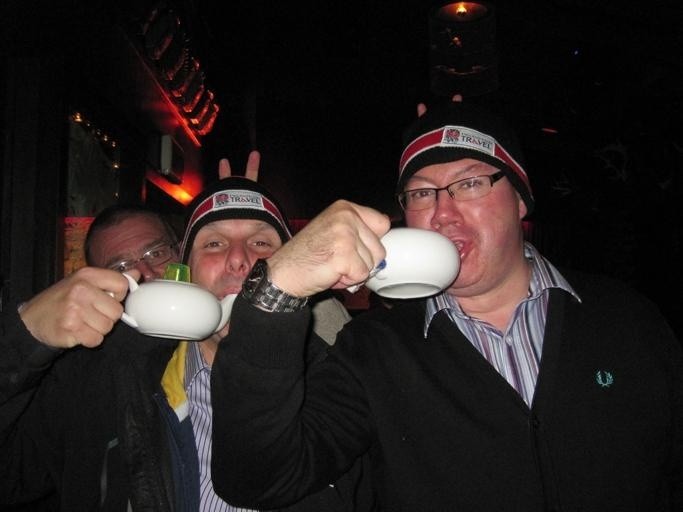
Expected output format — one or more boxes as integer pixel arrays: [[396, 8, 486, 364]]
[[84, 151, 261, 310], [210, 99, 682, 512], [1, 175, 375, 512]]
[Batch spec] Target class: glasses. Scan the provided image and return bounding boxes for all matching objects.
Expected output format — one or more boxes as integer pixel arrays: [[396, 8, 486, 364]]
[[398, 171, 504, 211], [106, 237, 177, 274]]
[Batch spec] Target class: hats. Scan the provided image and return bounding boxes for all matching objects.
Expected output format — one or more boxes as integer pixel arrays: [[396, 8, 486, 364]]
[[178, 174, 295, 265], [394, 102, 536, 221]]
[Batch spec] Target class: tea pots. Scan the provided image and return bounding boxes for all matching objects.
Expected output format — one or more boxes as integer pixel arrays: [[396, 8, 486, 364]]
[[364, 226, 462, 300], [107, 263, 239, 343]]
